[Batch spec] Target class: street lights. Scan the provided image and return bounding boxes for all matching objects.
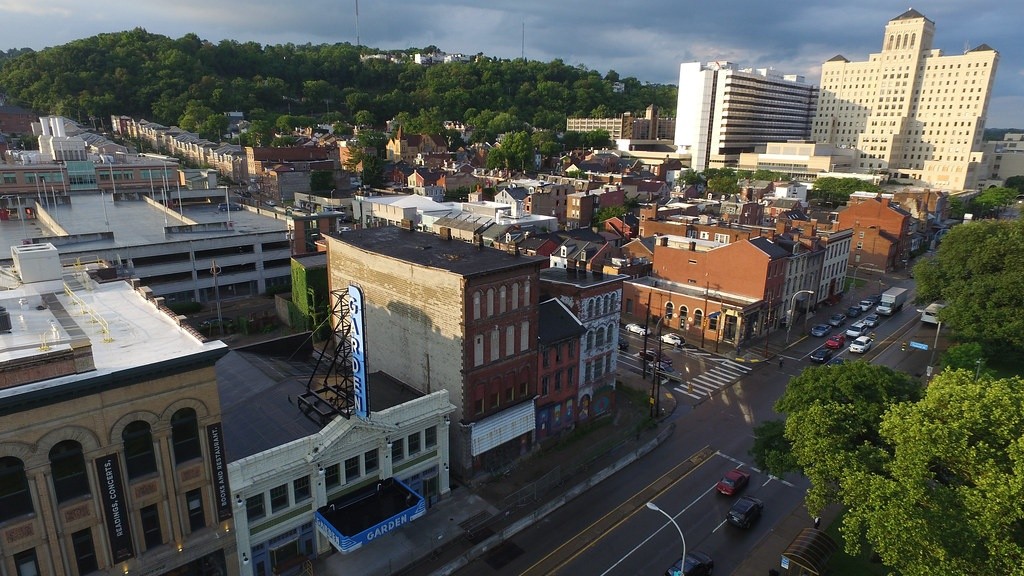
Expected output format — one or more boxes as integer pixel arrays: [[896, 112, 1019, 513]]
[[653, 312, 688, 383], [853, 262, 875, 289], [647, 501, 686, 576], [785, 290, 814, 347]]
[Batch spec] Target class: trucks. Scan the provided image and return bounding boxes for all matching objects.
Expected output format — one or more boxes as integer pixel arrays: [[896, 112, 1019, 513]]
[[875, 286, 908, 317]]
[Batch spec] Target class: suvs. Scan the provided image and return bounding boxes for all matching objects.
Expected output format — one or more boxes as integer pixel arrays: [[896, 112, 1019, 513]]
[[919, 303, 947, 324]]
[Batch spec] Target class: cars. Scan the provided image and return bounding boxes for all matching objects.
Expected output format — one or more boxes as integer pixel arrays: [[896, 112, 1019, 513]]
[[810, 294, 881, 363], [618, 323, 686, 383], [664, 550, 715, 576], [726, 494, 764, 530], [714, 469, 751, 497]]
